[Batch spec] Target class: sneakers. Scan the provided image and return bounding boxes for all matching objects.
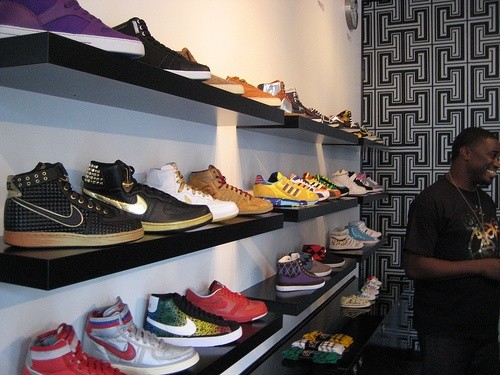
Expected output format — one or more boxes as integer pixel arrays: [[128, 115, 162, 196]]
[[188, 166, 275, 219], [251, 169, 387, 207], [4, 161, 143, 247], [144, 292, 243, 347], [276, 253, 326, 291], [110, 16, 211, 80], [145, 161, 240, 224], [260, 80, 381, 142], [17, 323, 128, 375], [301, 244, 345, 268], [288, 251, 333, 277], [82, 295, 200, 375], [1, 0, 145, 58], [226, 74, 282, 108], [176, 48, 245, 95], [185, 279, 269, 323], [82, 159, 211, 234]]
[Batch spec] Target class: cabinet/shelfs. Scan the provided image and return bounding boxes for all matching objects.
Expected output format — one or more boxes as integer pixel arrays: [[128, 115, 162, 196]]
[[0, 29, 392, 375]]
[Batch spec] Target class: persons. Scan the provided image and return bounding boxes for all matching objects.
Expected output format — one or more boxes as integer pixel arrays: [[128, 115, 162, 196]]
[[402, 127, 500, 375]]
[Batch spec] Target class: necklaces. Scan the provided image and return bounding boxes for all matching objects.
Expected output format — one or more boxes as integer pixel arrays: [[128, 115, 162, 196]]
[[449, 172, 486, 238]]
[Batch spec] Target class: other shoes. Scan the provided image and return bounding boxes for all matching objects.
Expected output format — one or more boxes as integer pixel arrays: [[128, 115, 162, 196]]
[[351, 220, 383, 239], [338, 274, 383, 307], [328, 228, 363, 250], [346, 224, 379, 245]]
